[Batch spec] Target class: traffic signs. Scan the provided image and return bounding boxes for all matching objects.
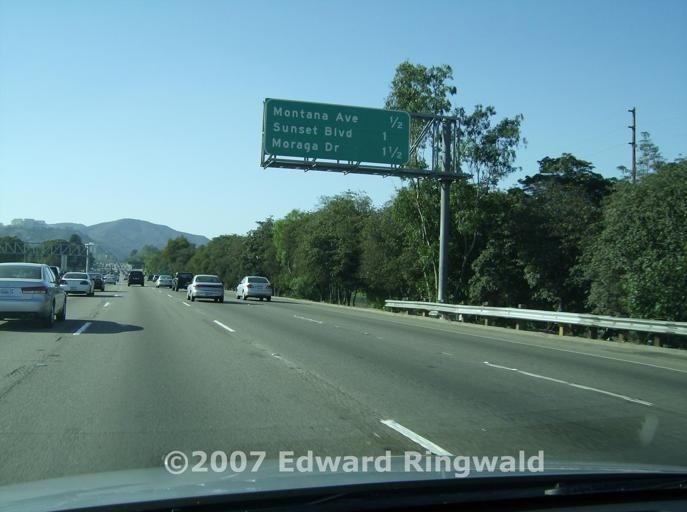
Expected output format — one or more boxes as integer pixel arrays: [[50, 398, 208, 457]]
[[262, 98, 410, 167]]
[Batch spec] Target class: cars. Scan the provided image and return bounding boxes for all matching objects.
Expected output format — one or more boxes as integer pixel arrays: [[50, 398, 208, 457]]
[[1, 456, 685, 511], [235, 275, 271, 303], [0, 262, 225, 326]]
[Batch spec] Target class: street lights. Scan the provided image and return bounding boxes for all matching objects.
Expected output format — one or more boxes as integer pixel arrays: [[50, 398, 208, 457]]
[[84, 241, 95, 273]]
